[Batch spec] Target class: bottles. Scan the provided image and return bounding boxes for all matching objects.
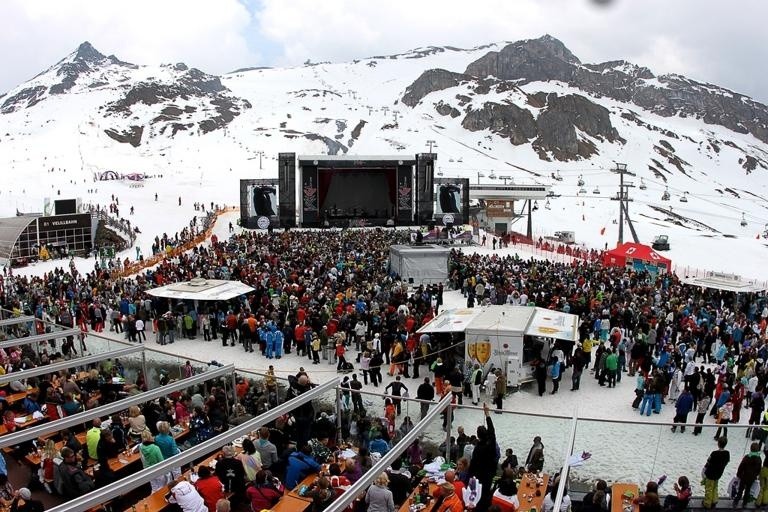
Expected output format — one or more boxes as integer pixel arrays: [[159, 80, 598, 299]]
[[130, 502, 150, 512], [409, 491, 420, 505], [523, 469, 541, 512]]
